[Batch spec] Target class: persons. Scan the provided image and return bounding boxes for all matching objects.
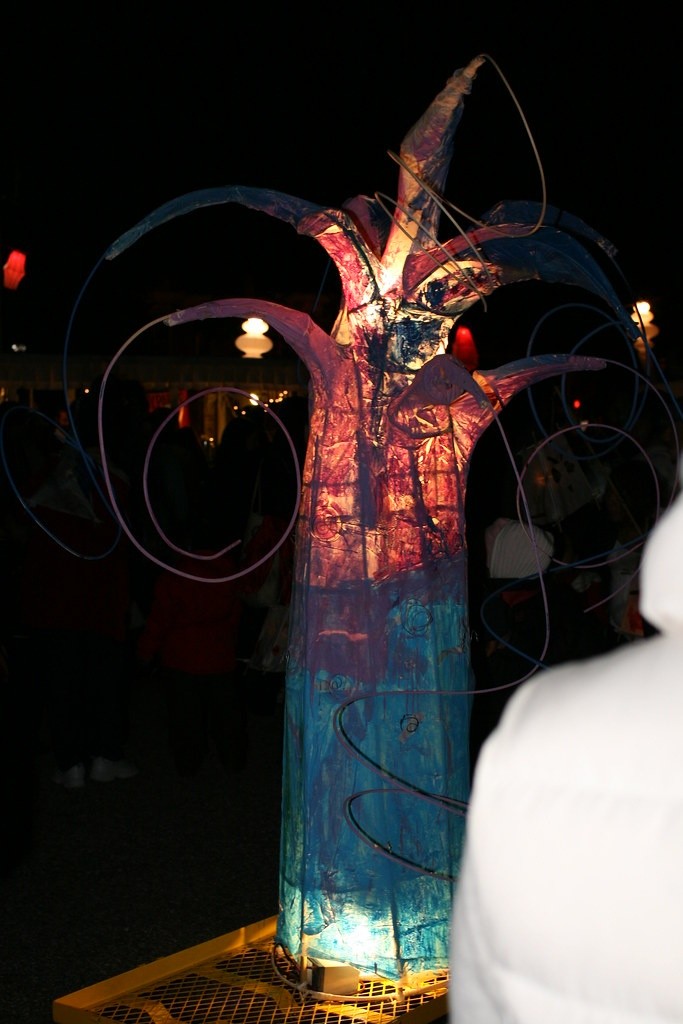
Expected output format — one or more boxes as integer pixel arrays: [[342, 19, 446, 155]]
[[0, 381, 310, 789], [467, 379, 683, 767]]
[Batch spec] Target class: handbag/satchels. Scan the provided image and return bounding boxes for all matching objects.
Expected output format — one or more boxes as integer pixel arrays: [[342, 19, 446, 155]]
[[237, 513, 280, 608], [252, 605, 289, 672]]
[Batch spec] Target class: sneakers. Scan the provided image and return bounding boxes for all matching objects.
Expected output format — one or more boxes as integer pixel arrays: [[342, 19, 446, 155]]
[[49, 763, 86, 788], [90, 756, 138, 781]]
